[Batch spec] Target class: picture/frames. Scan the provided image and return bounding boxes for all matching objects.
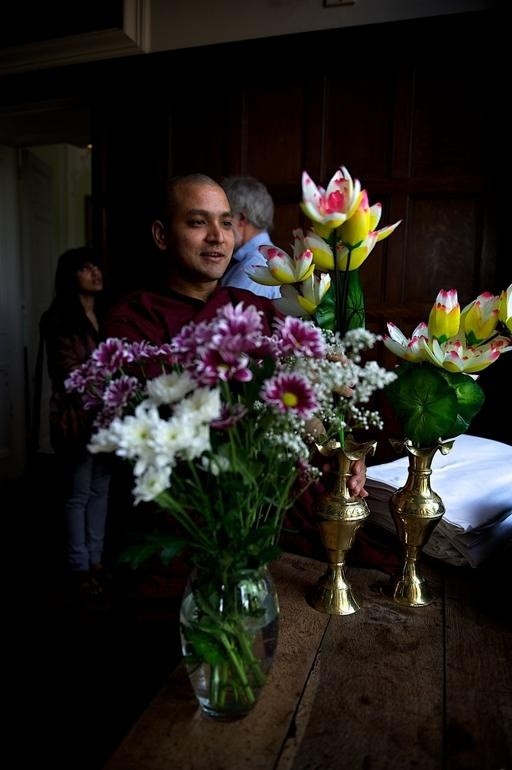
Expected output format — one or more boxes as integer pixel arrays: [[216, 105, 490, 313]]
[[1, 0, 151, 74]]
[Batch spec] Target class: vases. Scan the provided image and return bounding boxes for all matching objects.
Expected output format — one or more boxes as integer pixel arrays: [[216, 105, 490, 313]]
[[179, 556, 281, 724], [309, 433, 377, 616], [381, 435, 457, 608]]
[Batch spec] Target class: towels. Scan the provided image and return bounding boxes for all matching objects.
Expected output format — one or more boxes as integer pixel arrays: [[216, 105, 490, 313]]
[[362, 433, 511, 567]]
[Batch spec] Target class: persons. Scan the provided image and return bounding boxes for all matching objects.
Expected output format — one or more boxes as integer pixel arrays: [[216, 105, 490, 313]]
[[206, 172, 304, 314], [39, 246, 122, 603], [104, 174, 294, 659]]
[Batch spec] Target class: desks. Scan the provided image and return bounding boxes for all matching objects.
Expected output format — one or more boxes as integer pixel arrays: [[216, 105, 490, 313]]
[[99, 547, 512, 768]]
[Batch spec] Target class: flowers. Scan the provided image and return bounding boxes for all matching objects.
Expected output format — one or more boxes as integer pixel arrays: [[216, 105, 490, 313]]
[[64, 299, 399, 708], [378, 281, 511, 446], [244, 164, 407, 444]]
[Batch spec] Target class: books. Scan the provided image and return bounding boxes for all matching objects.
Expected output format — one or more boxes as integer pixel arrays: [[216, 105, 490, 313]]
[[358, 425, 512, 567]]
